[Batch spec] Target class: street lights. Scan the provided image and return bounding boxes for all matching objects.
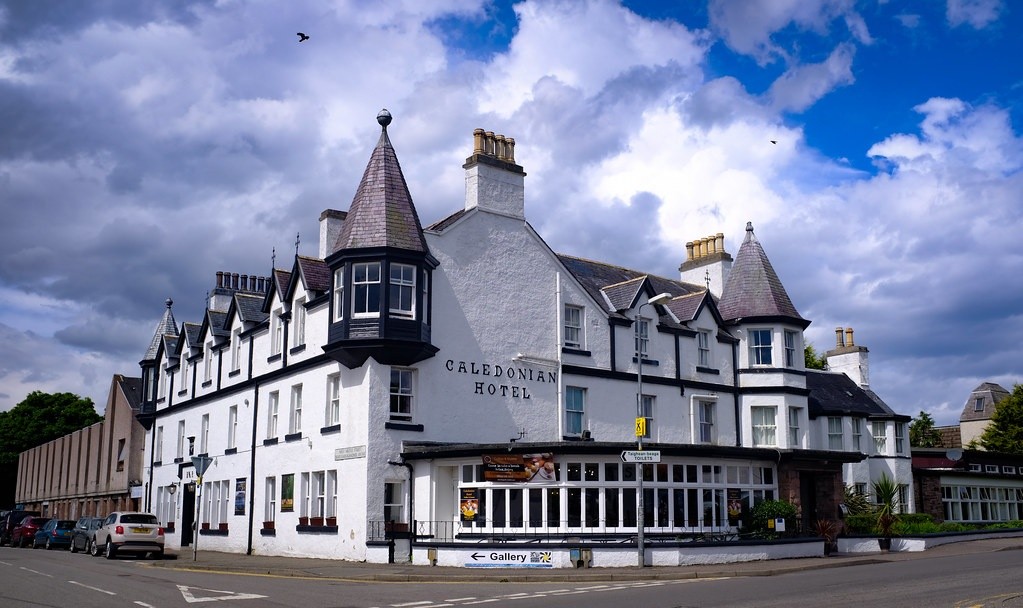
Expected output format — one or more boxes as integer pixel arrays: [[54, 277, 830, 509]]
[[638, 293, 673, 569]]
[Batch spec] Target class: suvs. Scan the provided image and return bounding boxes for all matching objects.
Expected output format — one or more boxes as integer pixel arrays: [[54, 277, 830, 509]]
[[0, 510, 42, 547], [90, 510, 165, 559]]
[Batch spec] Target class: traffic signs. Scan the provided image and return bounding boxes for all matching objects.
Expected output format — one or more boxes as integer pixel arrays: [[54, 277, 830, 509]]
[[464, 551, 552, 565], [619, 450, 661, 463]]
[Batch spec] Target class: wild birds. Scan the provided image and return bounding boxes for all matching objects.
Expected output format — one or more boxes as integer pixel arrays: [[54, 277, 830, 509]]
[[771, 141, 777, 144], [297, 33, 310, 42]]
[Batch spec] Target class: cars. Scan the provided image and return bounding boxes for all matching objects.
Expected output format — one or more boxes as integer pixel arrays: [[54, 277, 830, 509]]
[[9, 515, 52, 549], [70, 516, 105, 554], [32, 518, 78, 550]]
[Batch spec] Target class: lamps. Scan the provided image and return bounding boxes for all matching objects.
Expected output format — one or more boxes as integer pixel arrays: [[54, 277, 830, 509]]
[[168, 482, 180, 495], [582, 430, 591, 441], [188, 480, 196, 493]]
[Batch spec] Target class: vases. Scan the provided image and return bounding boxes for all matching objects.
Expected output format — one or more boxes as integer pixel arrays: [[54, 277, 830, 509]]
[[326, 517, 336, 526], [219, 523, 228, 530], [299, 517, 308, 526], [310, 517, 323, 526], [263, 521, 274, 529]]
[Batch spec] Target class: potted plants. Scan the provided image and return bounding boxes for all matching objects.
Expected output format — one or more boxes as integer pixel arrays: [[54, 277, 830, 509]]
[[872, 471, 907, 552], [817, 519, 842, 554]]
